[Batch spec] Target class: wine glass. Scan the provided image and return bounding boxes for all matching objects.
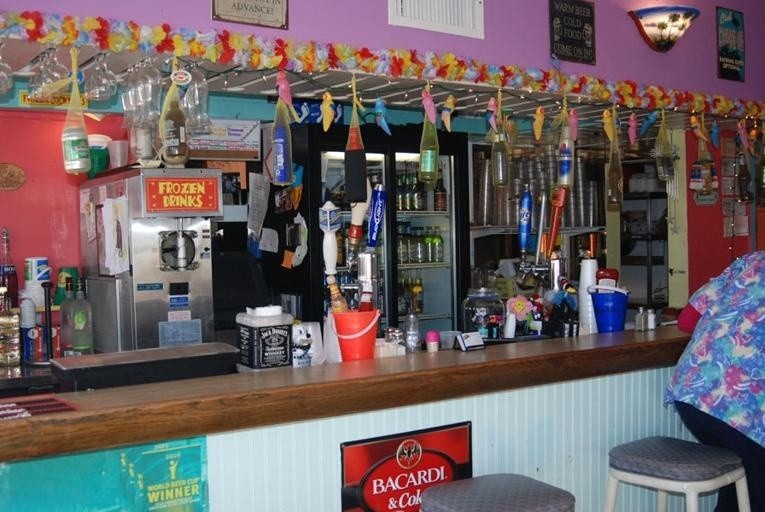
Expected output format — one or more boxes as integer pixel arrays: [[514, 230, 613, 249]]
[[0, 42, 214, 127], [162, 102, 193, 162]]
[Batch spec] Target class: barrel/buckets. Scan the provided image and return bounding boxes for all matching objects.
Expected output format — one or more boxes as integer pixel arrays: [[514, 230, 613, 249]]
[[332, 308, 382, 361], [585, 284, 632, 335]]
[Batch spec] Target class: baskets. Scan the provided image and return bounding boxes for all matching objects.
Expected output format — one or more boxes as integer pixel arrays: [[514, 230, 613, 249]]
[[489, 275, 542, 300]]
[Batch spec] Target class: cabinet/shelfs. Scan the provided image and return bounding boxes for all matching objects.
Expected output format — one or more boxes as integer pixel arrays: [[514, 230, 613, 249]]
[[287, 122, 470, 348], [468, 135, 668, 321]]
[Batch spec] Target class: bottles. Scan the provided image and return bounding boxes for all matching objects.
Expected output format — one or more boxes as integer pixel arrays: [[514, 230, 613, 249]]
[[394, 159, 449, 316], [267, 91, 295, 187], [689, 159, 719, 192], [556, 114, 578, 188], [405, 307, 422, 354], [333, 165, 384, 267], [635, 304, 657, 332], [418, 118, 440, 183], [358, 283, 372, 311], [489, 126, 510, 187], [0, 226, 92, 368], [606, 145, 625, 213], [325, 275, 351, 314]]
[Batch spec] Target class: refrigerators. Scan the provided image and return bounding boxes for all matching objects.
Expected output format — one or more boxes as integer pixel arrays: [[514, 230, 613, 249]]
[[394, 123, 470, 350], [262, 122, 394, 339]]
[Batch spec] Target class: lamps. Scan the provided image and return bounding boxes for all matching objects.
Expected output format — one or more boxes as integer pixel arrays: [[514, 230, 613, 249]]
[[627, 6, 702, 55]]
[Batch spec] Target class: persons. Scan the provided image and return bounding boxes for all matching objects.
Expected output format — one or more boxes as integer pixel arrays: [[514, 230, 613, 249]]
[[660, 246, 765, 512]]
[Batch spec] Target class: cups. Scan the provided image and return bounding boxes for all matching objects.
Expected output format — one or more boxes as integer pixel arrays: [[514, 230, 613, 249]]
[[578, 257, 598, 334], [474, 151, 600, 229], [107, 139, 129, 167]]
